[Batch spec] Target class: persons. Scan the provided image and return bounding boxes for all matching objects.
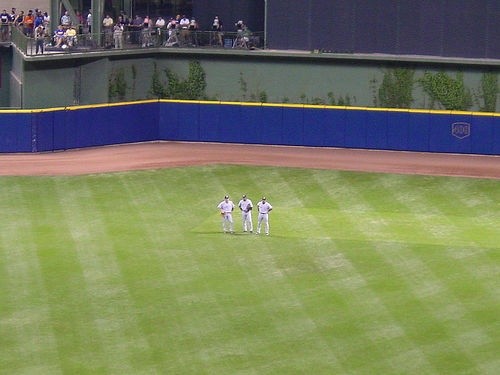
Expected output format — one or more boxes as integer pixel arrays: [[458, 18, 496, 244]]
[[61, 10, 72, 26], [256, 197, 272, 235], [238, 194, 254, 233], [232, 20, 244, 47], [143, 14, 201, 47], [35, 23, 45, 55], [217, 196, 235, 234], [86, 8, 91, 33], [75, 10, 83, 34], [212, 14, 224, 47], [0, 8, 50, 41], [48, 25, 76, 48], [103, 8, 144, 49]]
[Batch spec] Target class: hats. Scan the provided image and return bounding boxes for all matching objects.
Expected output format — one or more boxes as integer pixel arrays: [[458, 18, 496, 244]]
[[242, 194, 246, 198], [225, 195, 229, 199], [262, 196, 266, 200]]
